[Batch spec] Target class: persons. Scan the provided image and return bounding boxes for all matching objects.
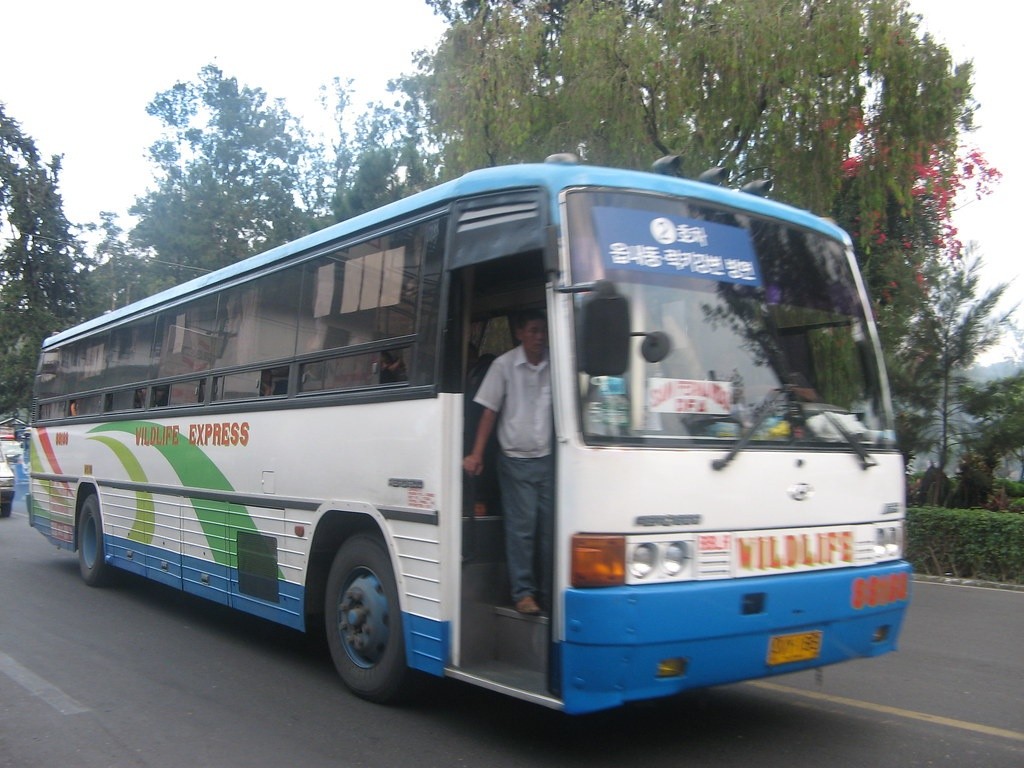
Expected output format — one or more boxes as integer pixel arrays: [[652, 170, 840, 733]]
[[733, 349, 820, 402], [337, 329, 403, 388], [463, 313, 555, 614], [260, 370, 272, 396]]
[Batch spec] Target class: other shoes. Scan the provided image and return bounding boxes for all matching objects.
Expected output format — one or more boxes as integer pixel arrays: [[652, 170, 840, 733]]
[[513, 595, 541, 613]]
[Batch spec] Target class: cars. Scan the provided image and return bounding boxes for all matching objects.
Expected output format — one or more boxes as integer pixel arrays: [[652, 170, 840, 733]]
[[0, 437, 15, 516]]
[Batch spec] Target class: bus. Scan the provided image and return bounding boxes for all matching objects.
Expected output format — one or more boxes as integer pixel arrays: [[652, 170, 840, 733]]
[[26, 153, 911, 716]]
